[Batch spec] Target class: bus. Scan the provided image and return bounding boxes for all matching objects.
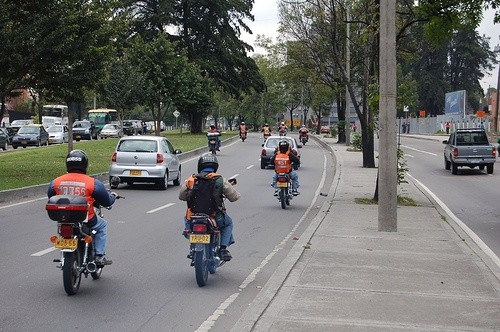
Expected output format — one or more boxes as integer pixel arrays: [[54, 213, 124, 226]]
[[87, 109, 121, 134], [41, 105, 69, 129]]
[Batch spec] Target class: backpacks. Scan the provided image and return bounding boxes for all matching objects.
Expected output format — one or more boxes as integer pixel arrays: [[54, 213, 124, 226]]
[[186, 172, 222, 215]]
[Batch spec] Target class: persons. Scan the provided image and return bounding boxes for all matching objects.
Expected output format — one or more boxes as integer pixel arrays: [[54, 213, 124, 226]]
[[179, 154, 241, 261], [478, 122, 484, 129], [261, 123, 272, 139], [206, 124, 221, 151], [47, 150, 118, 265], [87, 121, 97, 141], [239, 122, 248, 138], [353, 124, 357, 131], [279, 122, 287, 136], [441, 122, 444, 132], [446, 122, 450, 134], [400, 121, 411, 134], [406, 122, 410, 133], [272, 141, 301, 196], [208, 126, 221, 147], [401, 122, 407, 133], [131, 121, 149, 136], [273, 138, 297, 155], [443, 122, 447, 131], [298, 124, 309, 142]]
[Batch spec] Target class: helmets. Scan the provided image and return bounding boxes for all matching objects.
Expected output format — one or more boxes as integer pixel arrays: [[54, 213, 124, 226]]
[[66, 149, 88, 173], [281, 122, 284, 124], [241, 122, 245, 124], [279, 140, 289, 152], [302, 124, 306, 127], [265, 123, 269, 125], [197, 153, 219, 173]]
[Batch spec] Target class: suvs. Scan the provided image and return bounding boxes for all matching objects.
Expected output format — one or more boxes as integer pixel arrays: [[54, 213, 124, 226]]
[[135, 120, 146, 135], [10, 120, 34, 125], [72, 121, 97, 141]]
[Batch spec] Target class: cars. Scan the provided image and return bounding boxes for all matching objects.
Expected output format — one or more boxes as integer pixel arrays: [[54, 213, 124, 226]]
[[0, 128, 9, 150], [261, 136, 302, 170], [109, 136, 181, 190], [12, 124, 49, 149], [143, 122, 153, 133], [46, 125, 69, 145], [123, 121, 137, 136], [321, 126, 330, 134], [100, 124, 122, 139]]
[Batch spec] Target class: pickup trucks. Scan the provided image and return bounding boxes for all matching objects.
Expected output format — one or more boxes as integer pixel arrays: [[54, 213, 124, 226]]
[[442, 128, 497, 174]]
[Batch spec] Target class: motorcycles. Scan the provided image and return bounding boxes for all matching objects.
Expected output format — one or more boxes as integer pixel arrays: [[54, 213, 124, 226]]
[[45, 189, 126, 295], [239, 132, 247, 141], [209, 130, 222, 154], [274, 156, 300, 209], [301, 137, 308, 145], [279, 132, 286, 136], [182, 179, 237, 288]]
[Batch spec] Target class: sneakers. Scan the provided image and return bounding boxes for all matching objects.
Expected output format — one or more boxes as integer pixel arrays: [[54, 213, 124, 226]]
[[274, 191, 279, 196], [96, 256, 113, 266], [220, 248, 233, 260], [293, 190, 300, 195]]
